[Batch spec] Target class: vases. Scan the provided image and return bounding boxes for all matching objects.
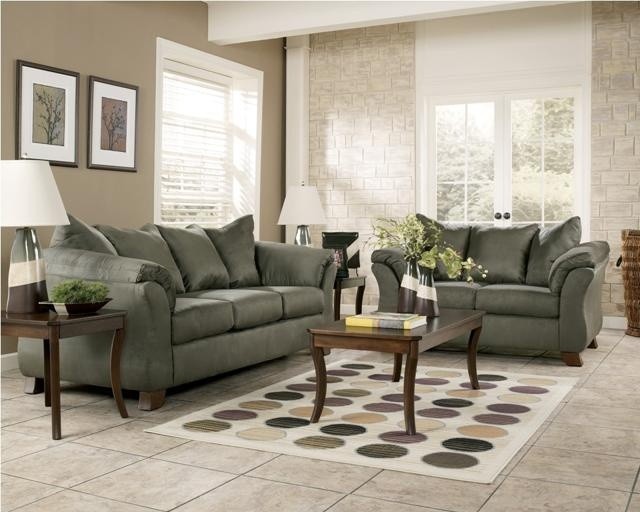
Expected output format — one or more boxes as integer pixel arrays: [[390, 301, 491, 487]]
[[397, 259, 417, 313], [414, 264, 441, 317]]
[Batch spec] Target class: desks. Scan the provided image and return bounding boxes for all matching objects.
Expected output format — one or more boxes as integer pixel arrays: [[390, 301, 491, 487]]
[[1, 307, 129, 442], [334, 274, 367, 322]]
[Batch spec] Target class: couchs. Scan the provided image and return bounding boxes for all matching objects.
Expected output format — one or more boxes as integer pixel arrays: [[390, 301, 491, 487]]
[[15, 238, 340, 412], [370, 239, 612, 367]]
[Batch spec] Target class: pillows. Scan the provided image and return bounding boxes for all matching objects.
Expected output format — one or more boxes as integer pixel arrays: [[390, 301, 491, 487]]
[[417, 213, 471, 280], [462, 222, 538, 285], [525, 216, 583, 289], [202, 213, 263, 288], [91, 221, 188, 294], [153, 222, 233, 294], [48, 213, 119, 256]]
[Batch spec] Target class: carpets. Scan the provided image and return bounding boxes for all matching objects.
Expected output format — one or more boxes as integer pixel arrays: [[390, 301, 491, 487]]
[[143, 356, 580, 487]]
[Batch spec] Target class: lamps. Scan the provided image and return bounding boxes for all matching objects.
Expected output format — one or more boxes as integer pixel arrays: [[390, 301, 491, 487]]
[[276, 182, 328, 246], [1, 159, 72, 316]]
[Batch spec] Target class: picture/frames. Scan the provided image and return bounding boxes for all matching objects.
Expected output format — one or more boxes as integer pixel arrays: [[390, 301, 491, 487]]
[[14, 58, 81, 169], [86, 76, 139, 173]]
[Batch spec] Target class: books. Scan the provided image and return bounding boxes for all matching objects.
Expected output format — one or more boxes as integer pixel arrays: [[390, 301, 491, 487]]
[[345, 312, 427, 329]]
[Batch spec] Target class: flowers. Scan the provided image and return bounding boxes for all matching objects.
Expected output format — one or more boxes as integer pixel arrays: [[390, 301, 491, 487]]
[[415, 242, 492, 284], [368, 210, 444, 259]]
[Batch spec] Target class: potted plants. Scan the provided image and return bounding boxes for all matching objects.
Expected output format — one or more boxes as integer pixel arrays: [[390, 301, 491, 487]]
[[38, 277, 115, 319]]
